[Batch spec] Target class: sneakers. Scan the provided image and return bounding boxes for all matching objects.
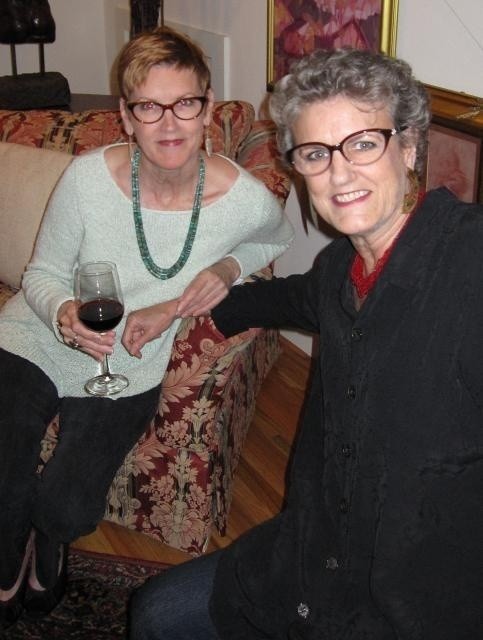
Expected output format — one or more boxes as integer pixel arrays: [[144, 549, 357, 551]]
[[0, 530, 28, 602], [27, 527, 66, 592]]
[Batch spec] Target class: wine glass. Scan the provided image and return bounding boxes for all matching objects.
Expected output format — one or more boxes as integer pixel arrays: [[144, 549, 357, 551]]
[[73, 263, 130, 396]]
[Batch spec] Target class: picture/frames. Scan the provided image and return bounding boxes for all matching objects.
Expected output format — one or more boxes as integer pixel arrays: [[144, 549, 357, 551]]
[[265, 0, 399, 93]]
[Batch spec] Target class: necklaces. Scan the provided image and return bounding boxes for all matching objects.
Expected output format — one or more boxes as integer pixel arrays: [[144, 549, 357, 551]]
[[131, 146, 205, 280]]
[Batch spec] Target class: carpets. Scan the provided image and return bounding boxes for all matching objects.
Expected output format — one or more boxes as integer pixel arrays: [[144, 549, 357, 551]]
[[0, 546, 176, 640]]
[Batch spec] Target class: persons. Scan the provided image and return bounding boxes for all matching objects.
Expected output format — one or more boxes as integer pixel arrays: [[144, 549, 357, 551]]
[[0, 25, 296, 628], [120, 44, 483, 639]]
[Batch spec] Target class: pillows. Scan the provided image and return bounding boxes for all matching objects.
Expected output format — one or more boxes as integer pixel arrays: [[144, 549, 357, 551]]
[[0, 141, 78, 291]]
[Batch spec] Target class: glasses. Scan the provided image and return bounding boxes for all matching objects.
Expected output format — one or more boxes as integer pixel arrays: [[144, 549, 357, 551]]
[[119, 93, 207, 126], [285, 127, 408, 175]]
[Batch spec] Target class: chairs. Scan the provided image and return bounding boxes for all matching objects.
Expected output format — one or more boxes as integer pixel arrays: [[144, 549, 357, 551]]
[[0, 101, 293, 558]]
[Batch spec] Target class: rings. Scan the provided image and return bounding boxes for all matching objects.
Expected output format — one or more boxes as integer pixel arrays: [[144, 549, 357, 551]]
[[141, 329, 144, 336], [72, 334, 79, 348]]
